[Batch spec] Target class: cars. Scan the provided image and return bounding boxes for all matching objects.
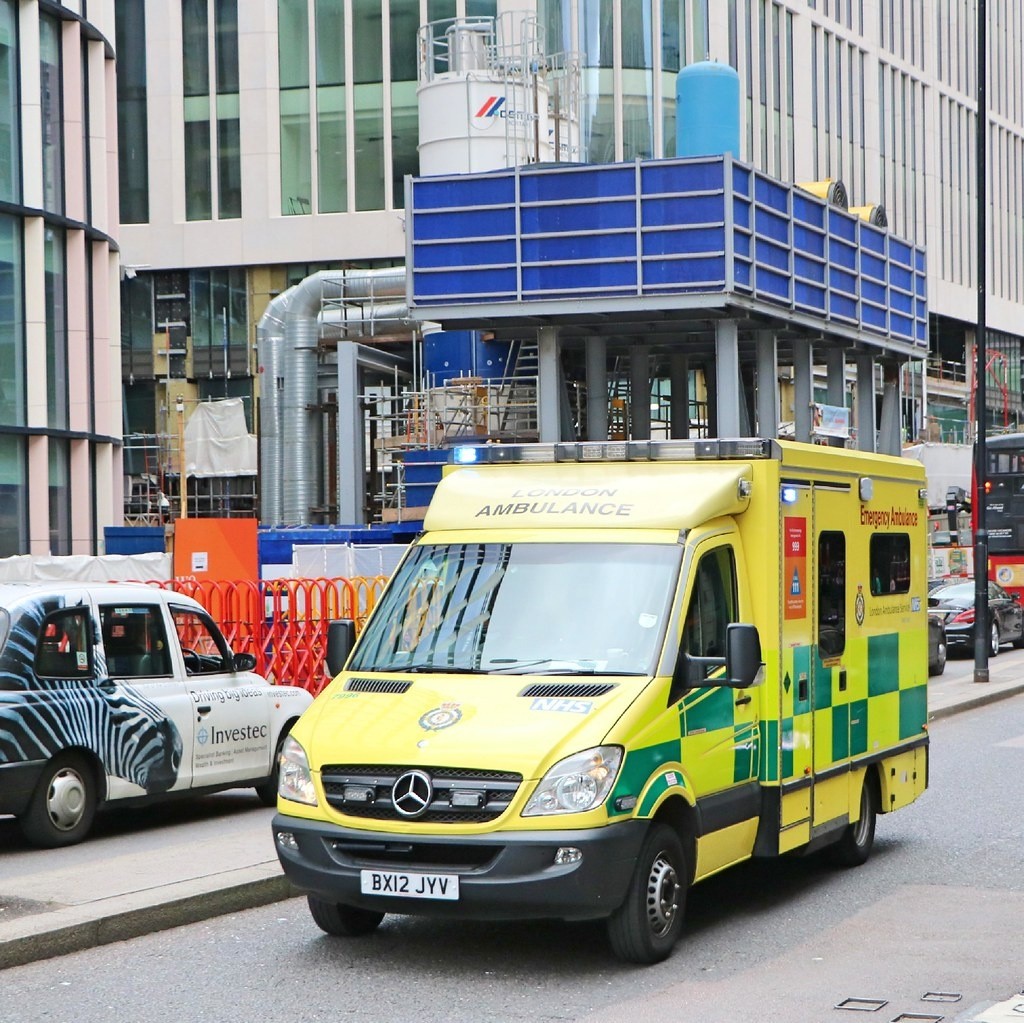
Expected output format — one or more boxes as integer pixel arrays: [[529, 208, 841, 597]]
[[0, 578, 316, 847], [927, 578, 1024, 657], [928, 597, 947, 676]]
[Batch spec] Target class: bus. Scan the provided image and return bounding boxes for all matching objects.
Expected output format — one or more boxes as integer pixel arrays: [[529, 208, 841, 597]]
[[969, 432, 1024, 621]]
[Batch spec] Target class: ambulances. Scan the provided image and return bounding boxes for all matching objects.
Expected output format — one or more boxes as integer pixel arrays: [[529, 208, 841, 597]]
[[271, 436, 932, 962]]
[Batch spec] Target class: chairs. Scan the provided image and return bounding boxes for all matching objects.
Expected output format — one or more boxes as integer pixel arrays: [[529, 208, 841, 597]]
[[41, 615, 79, 677], [114, 622, 164, 677]]
